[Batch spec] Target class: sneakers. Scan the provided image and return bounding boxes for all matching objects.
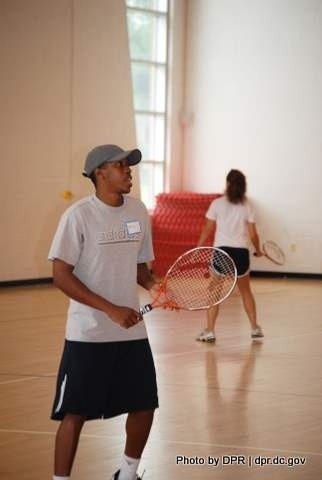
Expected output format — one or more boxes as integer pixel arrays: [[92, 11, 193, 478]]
[[196, 328, 216, 342], [251, 326, 264, 337]]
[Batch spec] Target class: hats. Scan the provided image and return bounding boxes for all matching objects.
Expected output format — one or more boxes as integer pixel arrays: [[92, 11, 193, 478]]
[[82, 145, 141, 178]]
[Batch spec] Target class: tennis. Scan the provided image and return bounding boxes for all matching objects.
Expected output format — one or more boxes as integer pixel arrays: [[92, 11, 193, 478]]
[[63, 191, 72, 200]]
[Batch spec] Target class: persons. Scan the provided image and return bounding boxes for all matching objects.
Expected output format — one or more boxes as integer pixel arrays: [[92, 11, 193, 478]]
[[49, 143, 177, 479], [195, 166, 268, 344]]
